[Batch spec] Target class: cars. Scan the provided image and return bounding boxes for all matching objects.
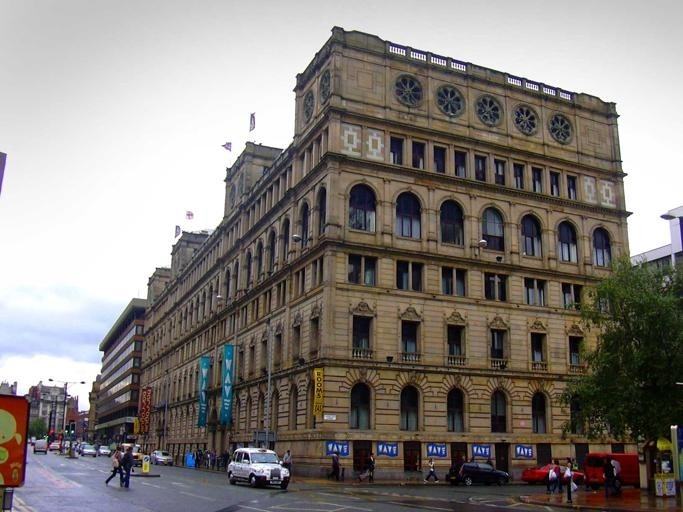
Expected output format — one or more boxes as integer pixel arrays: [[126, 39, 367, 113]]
[[31, 435, 112, 458], [150, 449, 174, 466], [520, 463, 586, 487]]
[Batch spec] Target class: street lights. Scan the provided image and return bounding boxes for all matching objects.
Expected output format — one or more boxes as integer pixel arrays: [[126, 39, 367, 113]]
[[49, 379, 86, 430]]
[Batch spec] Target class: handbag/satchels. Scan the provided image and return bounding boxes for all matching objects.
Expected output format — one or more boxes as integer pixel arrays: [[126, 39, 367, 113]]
[[563, 467, 571, 478]]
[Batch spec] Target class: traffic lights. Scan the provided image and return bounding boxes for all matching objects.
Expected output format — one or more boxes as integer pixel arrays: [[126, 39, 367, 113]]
[[66, 424, 71, 436], [59, 433, 64, 441], [70, 422, 75, 434], [49, 429, 56, 443]]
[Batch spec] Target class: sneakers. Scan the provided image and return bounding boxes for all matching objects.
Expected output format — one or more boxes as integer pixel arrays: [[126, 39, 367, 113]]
[[423, 479, 428, 482], [120, 479, 129, 488], [435, 480, 439, 483], [106, 481, 108, 486]]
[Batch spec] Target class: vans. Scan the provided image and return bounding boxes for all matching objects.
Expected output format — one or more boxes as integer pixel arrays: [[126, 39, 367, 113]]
[[117, 443, 145, 466], [581, 452, 639, 491]]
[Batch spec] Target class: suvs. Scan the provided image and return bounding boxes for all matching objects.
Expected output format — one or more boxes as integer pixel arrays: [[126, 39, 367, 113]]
[[227, 447, 290, 490], [458, 461, 510, 487]]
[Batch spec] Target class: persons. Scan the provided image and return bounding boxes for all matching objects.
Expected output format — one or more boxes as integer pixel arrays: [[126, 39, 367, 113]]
[[192, 447, 230, 473], [329, 452, 341, 481], [284, 449, 293, 476], [104, 446, 124, 488], [424, 456, 441, 485], [550, 455, 626, 495], [121, 447, 136, 489], [368, 452, 375, 482]]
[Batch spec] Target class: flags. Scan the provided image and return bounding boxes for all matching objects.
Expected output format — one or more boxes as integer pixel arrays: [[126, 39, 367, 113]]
[[251, 113, 255, 132], [221, 141, 231, 151], [175, 226, 181, 236], [187, 210, 195, 221]]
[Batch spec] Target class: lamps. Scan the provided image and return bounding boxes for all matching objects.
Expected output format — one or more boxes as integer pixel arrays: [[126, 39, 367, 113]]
[[216, 295, 231, 301], [292, 234, 313, 243], [470, 239, 487, 249]]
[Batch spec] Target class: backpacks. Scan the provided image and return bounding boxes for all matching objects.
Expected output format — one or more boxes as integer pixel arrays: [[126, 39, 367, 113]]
[[112, 455, 119, 468]]
